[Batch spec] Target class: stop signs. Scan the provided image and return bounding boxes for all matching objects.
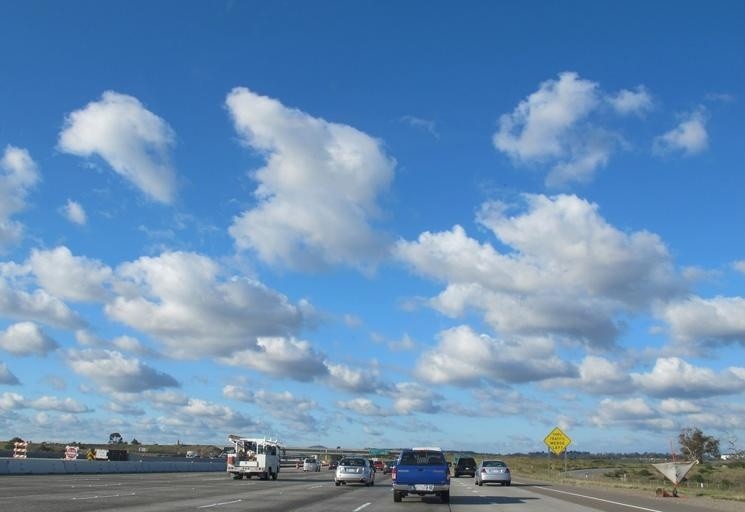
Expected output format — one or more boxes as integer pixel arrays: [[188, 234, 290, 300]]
[[66, 447, 76, 458]]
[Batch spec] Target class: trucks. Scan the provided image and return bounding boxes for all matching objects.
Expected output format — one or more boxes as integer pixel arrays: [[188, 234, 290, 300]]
[[93, 449, 129, 460], [186, 448, 201, 458], [227, 434, 285, 479]]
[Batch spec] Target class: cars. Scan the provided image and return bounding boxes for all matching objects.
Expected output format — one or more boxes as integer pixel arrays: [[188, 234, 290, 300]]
[[302, 448, 511, 501]]
